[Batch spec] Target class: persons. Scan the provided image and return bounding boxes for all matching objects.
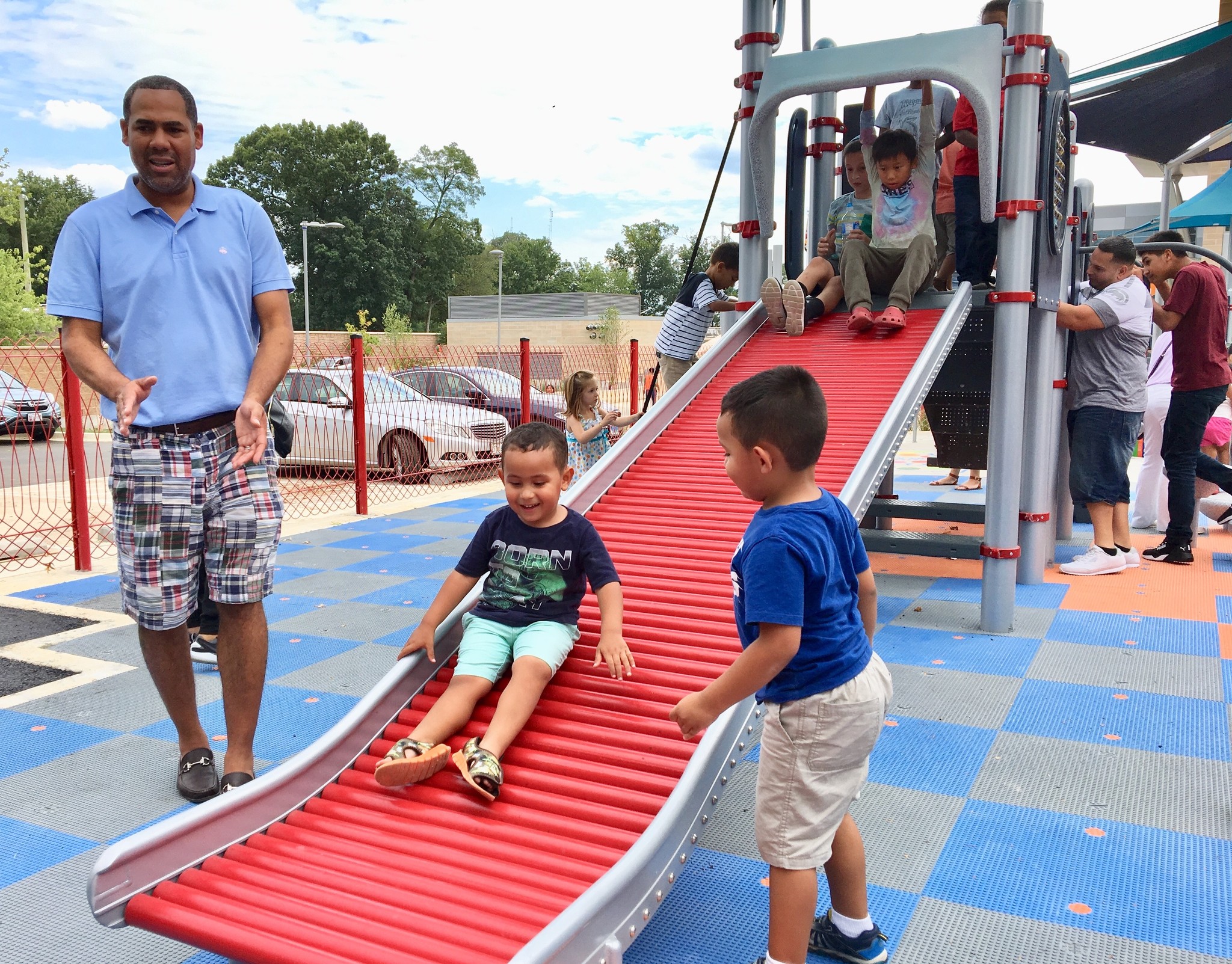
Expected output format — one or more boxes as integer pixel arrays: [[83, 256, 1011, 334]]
[[953, 89, 1005, 290], [670, 366, 893, 964], [546, 385, 555, 393], [374, 421, 639, 801], [929, 469, 984, 491], [184, 551, 219, 665], [760, 135, 879, 337], [874, 80, 957, 220], [1132, 261, 1232, 535], [46, 75, 296, 803], [931, 122, 964, 291], [641, 368, 658, 413], [655, 241, 739, 394], [838, 80, 937, 330], [1057, 235, 1153, 576], [561, 368, 645, 490], [981, 0, 1011, 79], [1132, 229, 1232, 564]]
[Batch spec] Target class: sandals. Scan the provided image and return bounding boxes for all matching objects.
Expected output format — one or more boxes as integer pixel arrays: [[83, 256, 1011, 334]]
[[954, 476, 983, 490], [929, 473, 959, 485], [374, 737, 451, 786], [452, 736, 503, 801]]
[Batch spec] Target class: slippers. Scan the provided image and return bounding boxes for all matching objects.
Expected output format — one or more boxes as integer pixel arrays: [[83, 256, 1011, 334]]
[[874, 307, 907, 328], [846, 306, 875, 331]]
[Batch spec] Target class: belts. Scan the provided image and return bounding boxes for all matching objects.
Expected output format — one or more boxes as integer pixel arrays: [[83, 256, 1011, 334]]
[[130, 409, 236, 437]]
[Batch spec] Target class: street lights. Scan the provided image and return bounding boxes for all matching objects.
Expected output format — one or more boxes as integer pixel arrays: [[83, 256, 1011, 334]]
[[300, 221, 345, 368], [720, 221, 733, 244], [489, 250, 505, 371]]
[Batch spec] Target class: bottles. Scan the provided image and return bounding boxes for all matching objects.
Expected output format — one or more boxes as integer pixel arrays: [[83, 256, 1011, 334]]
[[841, 202, 860, 246]]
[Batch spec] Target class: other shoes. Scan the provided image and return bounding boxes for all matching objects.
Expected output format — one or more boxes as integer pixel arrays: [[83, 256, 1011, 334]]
[[1149, 519, 1157, 528], [781, 279, 809, 336], [1217, 503, 1232, 524], [932, 278, 950, 292], [760, 277, 787, 329]]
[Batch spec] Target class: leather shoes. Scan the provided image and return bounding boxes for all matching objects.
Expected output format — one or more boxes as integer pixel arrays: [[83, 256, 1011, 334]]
[[176, 747, 220, 803], [221, 771, 256, 793]]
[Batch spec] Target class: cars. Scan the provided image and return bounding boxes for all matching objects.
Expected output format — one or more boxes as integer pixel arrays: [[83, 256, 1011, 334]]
[[265, 369, 511, 486], [390, 366, 621, 439], [0, 369, 61, 441], [313, 357, 352, 369]]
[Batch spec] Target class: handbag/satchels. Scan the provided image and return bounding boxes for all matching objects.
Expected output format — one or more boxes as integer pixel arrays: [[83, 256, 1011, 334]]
[[264, 394, 295, 458]]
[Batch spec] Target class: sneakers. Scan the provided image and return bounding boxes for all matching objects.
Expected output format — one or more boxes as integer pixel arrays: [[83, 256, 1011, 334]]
[[189, 633, 220, 664], [1142, 537, 1194, 562], [1059, 544, 1141, 576], [805, 907, 888, 964]]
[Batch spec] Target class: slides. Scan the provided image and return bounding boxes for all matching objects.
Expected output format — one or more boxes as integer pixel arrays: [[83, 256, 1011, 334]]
[[85, 270, 975, 964]]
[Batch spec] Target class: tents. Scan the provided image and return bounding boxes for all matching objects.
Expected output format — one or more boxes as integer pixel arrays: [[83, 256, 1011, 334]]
[[1121, 166, 1232, 234]]
